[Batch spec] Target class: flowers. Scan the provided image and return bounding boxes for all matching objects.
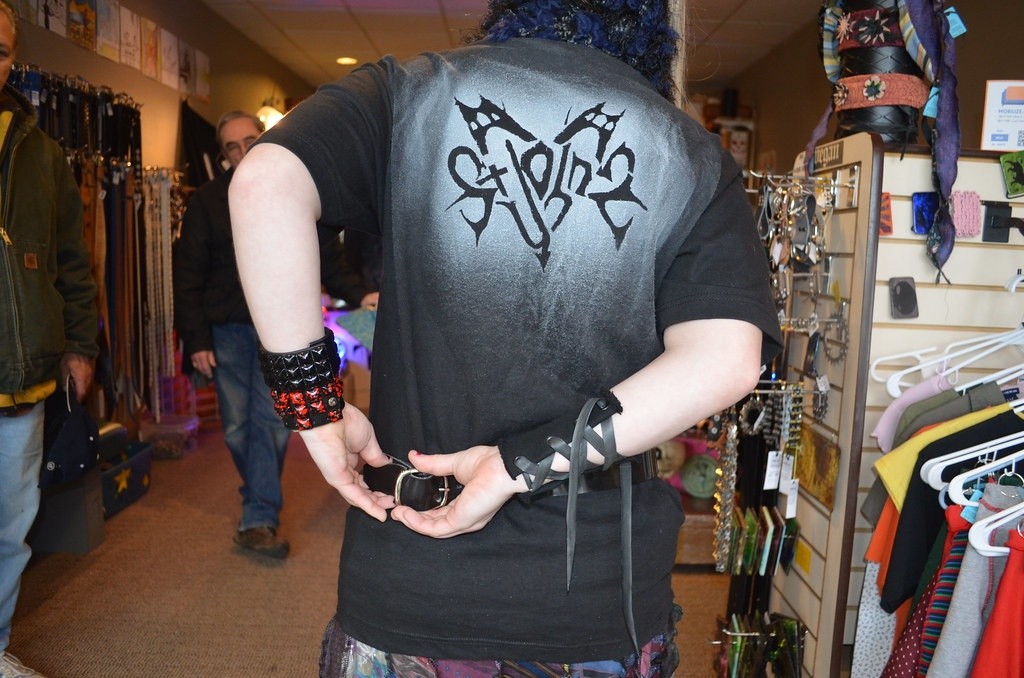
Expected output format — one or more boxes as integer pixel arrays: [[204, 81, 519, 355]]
[[834, 11, 856, 44], [863, 75, 887, 102], [833, 83, 849, 107], [858, 9, 891, 45]]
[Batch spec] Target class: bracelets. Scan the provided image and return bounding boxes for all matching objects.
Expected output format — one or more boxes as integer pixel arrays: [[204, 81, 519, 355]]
[[271, 376, 345, 431], [258, 326, 342, 391], [497, 386, 641, 656]]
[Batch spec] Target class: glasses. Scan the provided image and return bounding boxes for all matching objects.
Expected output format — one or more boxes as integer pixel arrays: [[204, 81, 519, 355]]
[[224, 141, 255, 157]]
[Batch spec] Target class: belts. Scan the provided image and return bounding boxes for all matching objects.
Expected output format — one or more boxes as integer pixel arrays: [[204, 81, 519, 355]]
[[362, 449, 656, 509], [8, 61, 173, 423]]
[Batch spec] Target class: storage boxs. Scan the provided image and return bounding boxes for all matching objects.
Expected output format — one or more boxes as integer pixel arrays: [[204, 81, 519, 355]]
[[102, 441, 154, 519], [139, 416, 200, 462]]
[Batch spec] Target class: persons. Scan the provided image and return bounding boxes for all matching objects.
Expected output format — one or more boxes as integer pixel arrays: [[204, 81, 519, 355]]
[[0, 0, 100, 678], [177, 109, 380, 557], [42, 0, 54, 30], [228, 0, 786, 678]]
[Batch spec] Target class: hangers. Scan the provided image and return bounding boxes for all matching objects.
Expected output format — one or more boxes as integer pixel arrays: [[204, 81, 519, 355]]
[[868, 276, 1024, 556]]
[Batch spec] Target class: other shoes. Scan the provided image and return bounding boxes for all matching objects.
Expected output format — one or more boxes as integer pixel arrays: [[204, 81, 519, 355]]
[[233, 528, 290, 560], [0, 650, 45, 678]]
